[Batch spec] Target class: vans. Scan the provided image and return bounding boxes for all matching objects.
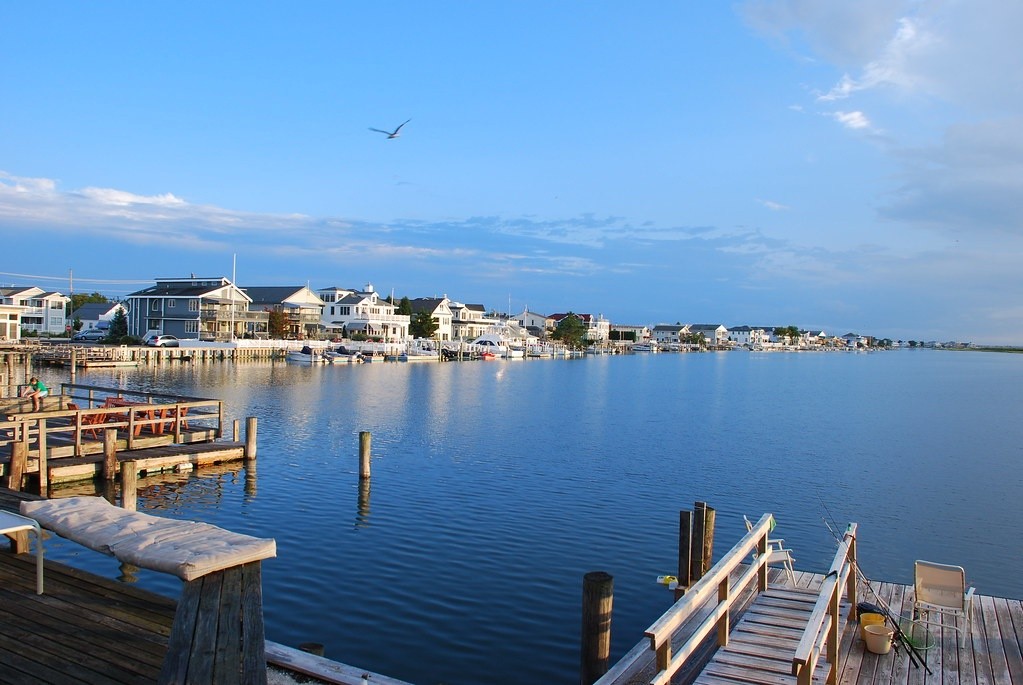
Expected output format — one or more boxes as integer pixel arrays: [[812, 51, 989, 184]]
[[73, 330, 105, 340]]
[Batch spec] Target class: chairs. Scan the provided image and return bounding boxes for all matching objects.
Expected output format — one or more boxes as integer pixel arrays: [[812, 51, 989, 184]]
[[67, 402, 98, 440], [908, 558, 977, 648], [159, 401, 190, 431], [742, 512, 798, 589], [97, 395, 125, 429]]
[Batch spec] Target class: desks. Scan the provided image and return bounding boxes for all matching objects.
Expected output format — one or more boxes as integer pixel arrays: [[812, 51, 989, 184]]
[[111, 401, 167, 428]]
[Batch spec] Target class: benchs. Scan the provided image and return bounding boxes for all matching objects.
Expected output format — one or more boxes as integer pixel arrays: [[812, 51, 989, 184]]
[[137, 411, 180, 431], [104, 412, 149, 436]]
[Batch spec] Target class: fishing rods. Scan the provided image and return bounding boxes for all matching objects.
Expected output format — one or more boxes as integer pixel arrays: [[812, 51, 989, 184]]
[[820, 515, 934, 676], [816, 492, 919, 670]]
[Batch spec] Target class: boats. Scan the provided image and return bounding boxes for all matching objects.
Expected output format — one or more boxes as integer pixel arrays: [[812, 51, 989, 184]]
[[365, 354, 385, 362], [326, 347, 365, 363], [287, 345, 328, 362], [440, 332, 760, 362], [388, 351, 408, 361]]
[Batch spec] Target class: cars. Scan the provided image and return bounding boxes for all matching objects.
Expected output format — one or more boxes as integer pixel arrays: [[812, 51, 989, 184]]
[[148, 334, 180, 347]]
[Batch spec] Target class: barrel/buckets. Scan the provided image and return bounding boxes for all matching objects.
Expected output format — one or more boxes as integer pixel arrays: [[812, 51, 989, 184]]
[[864, 624, 894, 654], [859, 612, 885, 640]]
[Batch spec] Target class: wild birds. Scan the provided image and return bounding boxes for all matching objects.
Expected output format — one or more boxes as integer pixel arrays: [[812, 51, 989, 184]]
[[366, 117, 413, 139]]
